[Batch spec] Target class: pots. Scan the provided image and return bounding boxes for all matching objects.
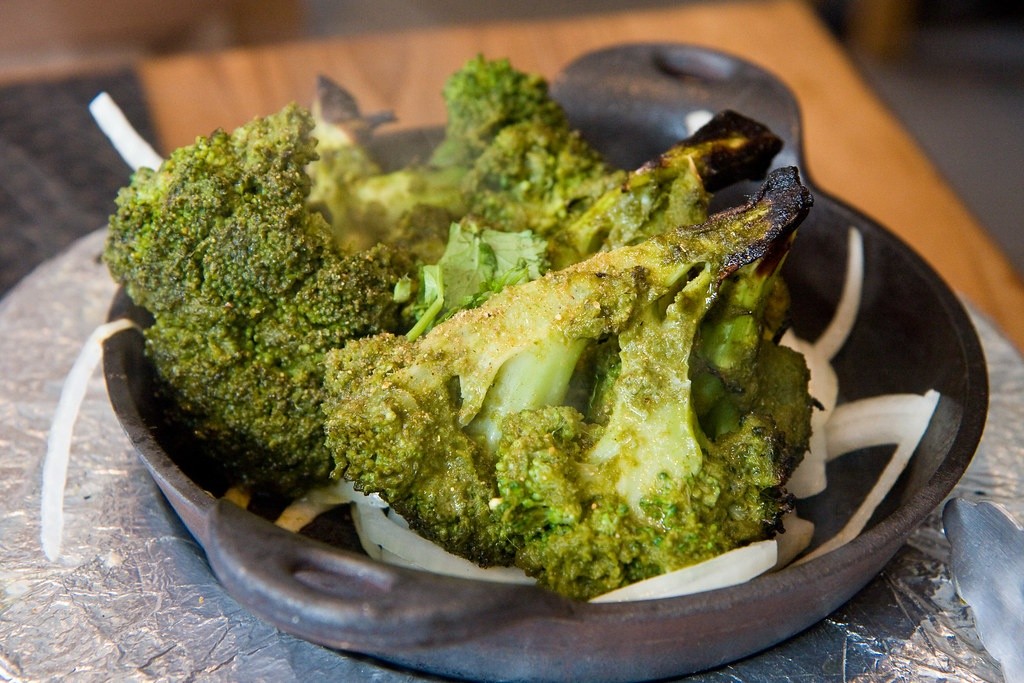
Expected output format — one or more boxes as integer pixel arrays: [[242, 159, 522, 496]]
[[98, 40, 992, 683]]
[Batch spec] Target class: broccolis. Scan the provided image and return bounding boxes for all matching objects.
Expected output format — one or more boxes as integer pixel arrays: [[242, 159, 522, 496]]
[[104, 53, 830, 604]]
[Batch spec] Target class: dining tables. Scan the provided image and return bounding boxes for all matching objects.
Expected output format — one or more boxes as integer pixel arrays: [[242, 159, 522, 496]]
[[0, 0, 1023, 360]]
